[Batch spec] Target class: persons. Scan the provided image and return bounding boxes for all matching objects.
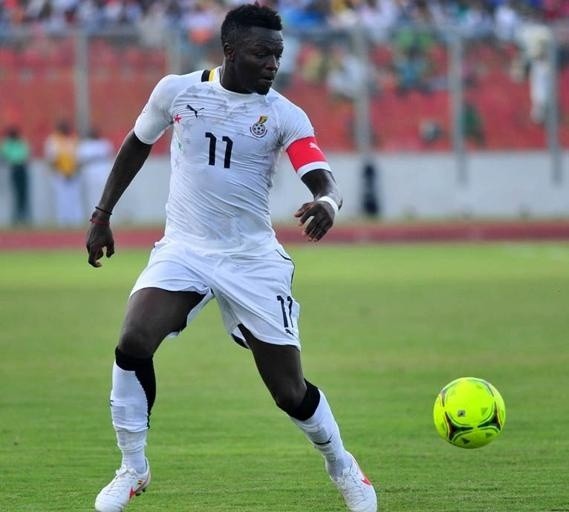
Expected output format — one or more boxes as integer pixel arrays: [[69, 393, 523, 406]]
[[0, 0, 232, 73], [78, 117, 116, 211], [2, 123, 38, 231], [84, 5, 378, 510], [41, 113, 81, 225], [275, 0, 569, 151]]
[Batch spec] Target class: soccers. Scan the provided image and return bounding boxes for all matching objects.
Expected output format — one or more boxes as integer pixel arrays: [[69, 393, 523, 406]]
[[433, 377, 506, 448]]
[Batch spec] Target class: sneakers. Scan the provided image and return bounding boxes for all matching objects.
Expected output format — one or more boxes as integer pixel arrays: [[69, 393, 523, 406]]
[[324, 451, 377, 511], [94, 457, 152, 511]]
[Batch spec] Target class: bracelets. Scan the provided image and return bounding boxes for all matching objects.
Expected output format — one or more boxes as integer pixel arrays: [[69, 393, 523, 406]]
[[95, 205, 112, 215], [90, 218, 112, 224], [314, 191, 352, 216]]
